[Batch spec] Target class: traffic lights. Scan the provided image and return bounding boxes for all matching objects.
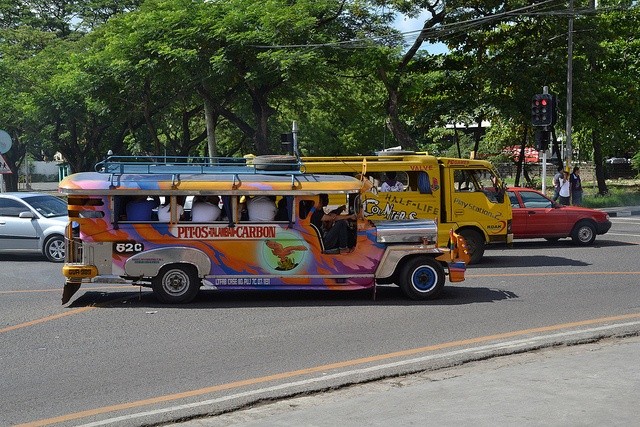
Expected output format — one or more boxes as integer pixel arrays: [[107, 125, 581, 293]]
[[541, 98, 549, 123], [533, 96, 540, 124]]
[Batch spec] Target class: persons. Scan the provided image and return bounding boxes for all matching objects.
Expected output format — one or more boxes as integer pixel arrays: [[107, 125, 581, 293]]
[[552, 166, 564, 201], [559, 171, 571, 206], [305, 193, 357, 254], [158, 196, 185, 222], [381, 171, 404, 192], [569, 166, 583, 206], [126, 195, 161, 221], [247, 195, 278, 222], [189, 196, 222, 221]]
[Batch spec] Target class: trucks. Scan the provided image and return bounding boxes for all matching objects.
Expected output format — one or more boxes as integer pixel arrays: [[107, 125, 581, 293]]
[[240, 153, 514, 265]]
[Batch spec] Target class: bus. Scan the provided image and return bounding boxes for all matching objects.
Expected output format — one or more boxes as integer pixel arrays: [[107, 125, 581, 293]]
[[60, 156, 471, 300]]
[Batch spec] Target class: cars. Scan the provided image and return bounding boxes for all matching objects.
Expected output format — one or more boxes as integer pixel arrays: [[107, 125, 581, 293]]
[[483, 187, 612, 245], [1, 192, 70, 263]]
